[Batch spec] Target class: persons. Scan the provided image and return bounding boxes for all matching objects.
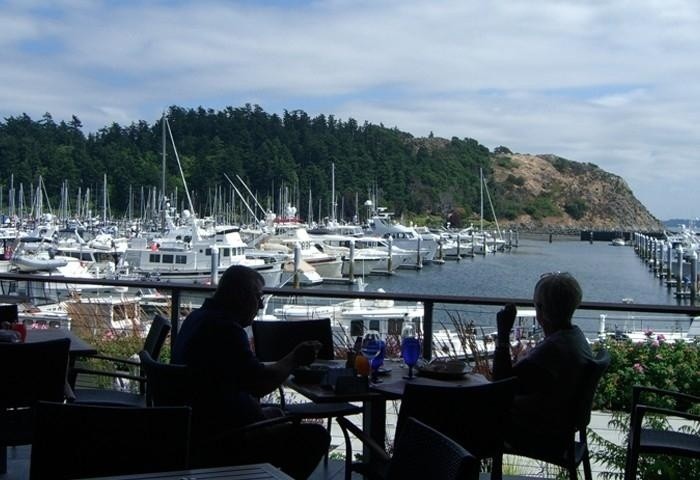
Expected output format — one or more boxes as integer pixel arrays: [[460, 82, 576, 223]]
[[167, 265, 331, 480], [462, 272, 593, 480]]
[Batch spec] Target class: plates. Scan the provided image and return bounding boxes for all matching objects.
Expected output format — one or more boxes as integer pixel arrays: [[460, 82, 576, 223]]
[[418, 364, 473, 378], [377, 366, 393, 374], [291, 366, 310, 377]]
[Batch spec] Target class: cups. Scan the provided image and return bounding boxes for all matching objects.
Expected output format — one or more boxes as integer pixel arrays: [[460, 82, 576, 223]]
[[355, 356, 370, 377], [11, 323, 27, 341]]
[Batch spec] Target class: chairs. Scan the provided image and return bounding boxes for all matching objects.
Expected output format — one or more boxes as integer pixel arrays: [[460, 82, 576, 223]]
[[0, 305, 699, 480]]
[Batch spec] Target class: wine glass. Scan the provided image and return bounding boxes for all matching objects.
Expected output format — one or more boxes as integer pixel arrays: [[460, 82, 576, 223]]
[[399, 321, 420, 380], [360, 330, 386, 383]]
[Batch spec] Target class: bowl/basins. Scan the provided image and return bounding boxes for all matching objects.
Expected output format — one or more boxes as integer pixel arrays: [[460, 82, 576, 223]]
[[447, 360, 466, 373]]
